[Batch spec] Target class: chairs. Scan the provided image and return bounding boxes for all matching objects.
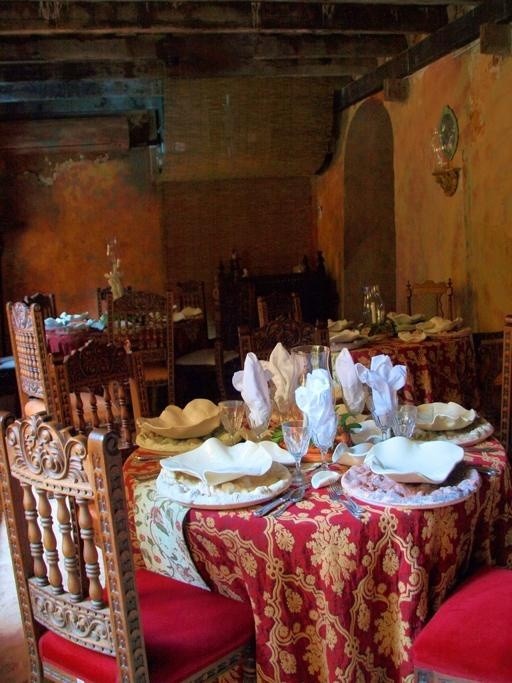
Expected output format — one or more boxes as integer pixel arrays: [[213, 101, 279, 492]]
[[412, 567, 510, 682], [173, 275, 248, 402], [2, 255, 511, 376], [4, 300, 123, 430], [101, 284, 173, 408], [1, 411, 254, 682], [47, 338, 151, 551]]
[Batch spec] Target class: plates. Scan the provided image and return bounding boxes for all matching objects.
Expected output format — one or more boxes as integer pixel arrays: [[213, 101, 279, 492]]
[[387, 310, 462, 343], [137, 398, 221, 439], [159, 436, 295, 485], [43, 311, 92, 329], [334, 435, 466, 486], [416, 401, 477, 431], [327, 318, 359, 344]]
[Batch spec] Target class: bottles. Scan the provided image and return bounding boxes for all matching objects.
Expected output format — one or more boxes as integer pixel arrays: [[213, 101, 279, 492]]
[[362, 284, 385, 326]]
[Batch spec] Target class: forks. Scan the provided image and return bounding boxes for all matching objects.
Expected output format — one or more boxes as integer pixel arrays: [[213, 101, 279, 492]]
[[328, 481, 366, 521]]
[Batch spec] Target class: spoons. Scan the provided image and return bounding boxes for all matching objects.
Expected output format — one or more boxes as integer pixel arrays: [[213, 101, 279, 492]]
[[272, 486, 307, 518]]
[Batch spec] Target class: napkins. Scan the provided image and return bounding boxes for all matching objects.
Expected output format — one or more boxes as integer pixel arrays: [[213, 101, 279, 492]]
[[232, 352, 275, 428], [260, 342, 300, 405], [295, 366, 338, 452], [335, 347, 368, 412], [354, 353, 409, 417]]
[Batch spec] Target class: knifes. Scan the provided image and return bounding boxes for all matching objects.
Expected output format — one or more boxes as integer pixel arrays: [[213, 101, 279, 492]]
[[254, 478, 309, 517]]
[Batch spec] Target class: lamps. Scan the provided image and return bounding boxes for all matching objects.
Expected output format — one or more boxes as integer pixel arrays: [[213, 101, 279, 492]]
[[431, 105, 461, 198]]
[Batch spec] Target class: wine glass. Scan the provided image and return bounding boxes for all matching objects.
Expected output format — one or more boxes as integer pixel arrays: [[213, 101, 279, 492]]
[[283, 344, 337, 482], [216, 391, 299, 443], [342, 380, 415, 443]]
